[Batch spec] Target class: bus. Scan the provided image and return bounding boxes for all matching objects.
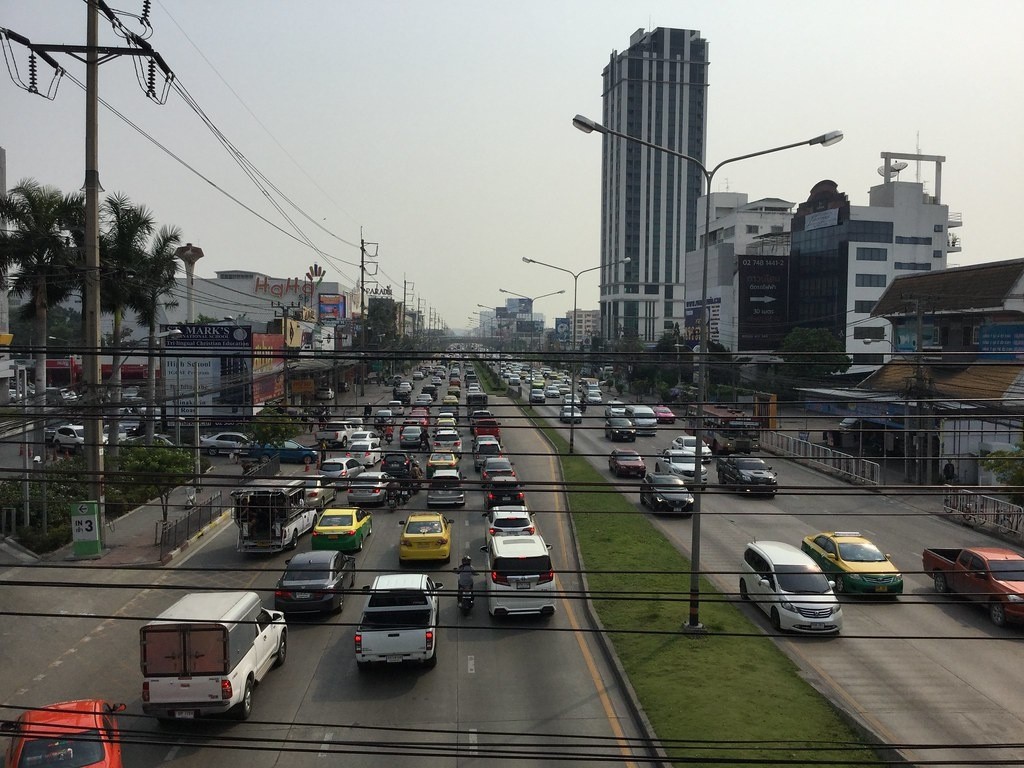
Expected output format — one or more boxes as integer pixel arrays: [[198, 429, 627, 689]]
[[684, 400, 760, 454]]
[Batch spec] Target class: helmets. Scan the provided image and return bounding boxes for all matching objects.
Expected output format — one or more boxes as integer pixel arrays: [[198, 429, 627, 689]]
[[462, 554, 471, 564]]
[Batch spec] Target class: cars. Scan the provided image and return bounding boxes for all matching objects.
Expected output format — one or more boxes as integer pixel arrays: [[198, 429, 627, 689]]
[[397, 510, 454, 564], [1, 698, 126, 768], [311, 507, 372, 551], [275, 550, 356, 618], [10, 343, 781, 517], [481, 504, 536, 544], [804, 528, 903, 601]]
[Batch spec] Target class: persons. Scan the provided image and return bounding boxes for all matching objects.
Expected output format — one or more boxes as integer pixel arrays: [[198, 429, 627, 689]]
[[232, 441, 240, 464], [943, 459, 955, 484], [376, 418, 394, 441], [580, 398, 587, 410], [300, 402, 331, 433], [451, 556, 479, 608], [418, 428, 431, 451], [319, 438, 327, 461], [386, 461, 425, 506], [364, 403, 372, 415], [238, 497, 304, 539]]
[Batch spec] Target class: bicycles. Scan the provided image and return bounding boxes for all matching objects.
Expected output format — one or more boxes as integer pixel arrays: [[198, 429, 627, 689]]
[[944, 489, 1013, 534]]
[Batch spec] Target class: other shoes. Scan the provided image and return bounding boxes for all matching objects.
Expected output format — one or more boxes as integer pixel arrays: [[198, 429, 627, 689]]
[[471, 601, 474, 603], [458, 602, 462, 607]]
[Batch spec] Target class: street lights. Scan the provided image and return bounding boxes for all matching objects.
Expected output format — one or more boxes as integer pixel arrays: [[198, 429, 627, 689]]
[[284, 339, 323, 413], [361, 334, 386, 397], [675, 345, 709, 403], [572, 111, 845, 631], [473, 311, 509, 374], [864, 338, 923, 484], [521, 257, 632, 454], [500, 288, 566, 409], [80, 329, 183, 547], [478, 304, 524, 386], [224, 316, 245, 433]]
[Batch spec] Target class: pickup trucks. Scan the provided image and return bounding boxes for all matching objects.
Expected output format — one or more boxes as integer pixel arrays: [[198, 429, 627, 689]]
[[354, 572, 445, 670], [924, 546, 1024, 626]]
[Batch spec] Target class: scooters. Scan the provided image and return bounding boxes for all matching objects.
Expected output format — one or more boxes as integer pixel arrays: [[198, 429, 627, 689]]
[[450, 569, 479, 612]]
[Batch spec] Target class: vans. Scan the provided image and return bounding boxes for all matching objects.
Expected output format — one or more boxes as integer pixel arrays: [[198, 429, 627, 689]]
[[739, 541, 841, 635], [480, 535, 557, 617]]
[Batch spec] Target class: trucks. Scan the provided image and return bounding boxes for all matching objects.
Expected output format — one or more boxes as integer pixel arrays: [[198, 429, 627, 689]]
[[230, 478, 317, 553], [136, 591, 288, 726]]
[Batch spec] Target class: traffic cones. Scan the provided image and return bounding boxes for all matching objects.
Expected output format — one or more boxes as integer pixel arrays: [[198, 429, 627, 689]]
[[28, 444, 31, 457], [18, 444, 23, 456]]
